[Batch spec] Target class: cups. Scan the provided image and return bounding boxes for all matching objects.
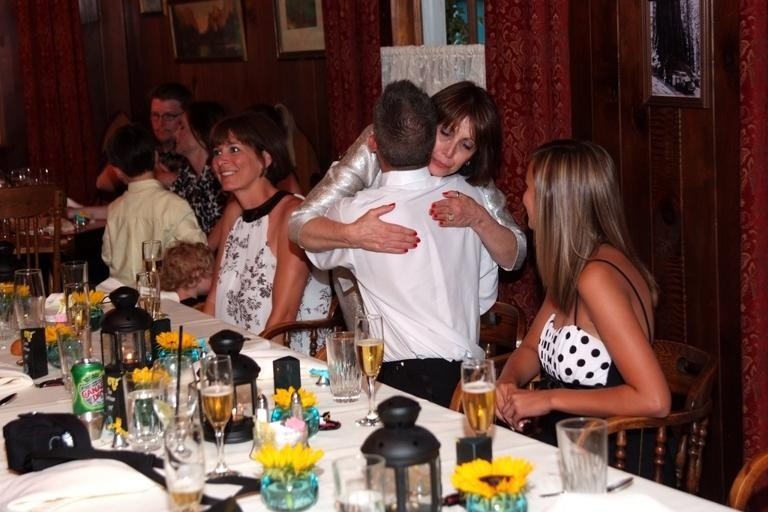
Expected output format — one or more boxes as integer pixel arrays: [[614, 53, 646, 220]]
[[324, 330, 363, 404], [556, 416, 608, 494], [77, 411, 106, 441], [61, 260, 89, 299], [333, 452, 387, 510], [121, 370, 161, 453]]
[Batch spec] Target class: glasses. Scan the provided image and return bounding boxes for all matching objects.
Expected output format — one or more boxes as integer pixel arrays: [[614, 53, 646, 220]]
[[152, 112, 184, 121]]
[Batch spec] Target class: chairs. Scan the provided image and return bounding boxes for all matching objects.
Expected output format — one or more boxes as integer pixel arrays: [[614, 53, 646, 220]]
[[0, 167, 315, 295], [732, 451, 768, 510], [571, 339, 725, 492], [314, 298, 536, 433], [181, 261, 351, 366]]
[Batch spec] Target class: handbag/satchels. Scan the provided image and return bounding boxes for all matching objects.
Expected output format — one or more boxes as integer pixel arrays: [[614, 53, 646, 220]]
[[3, 411, 91, 474]]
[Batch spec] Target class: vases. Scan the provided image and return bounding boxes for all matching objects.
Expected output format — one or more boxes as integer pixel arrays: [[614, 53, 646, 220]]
[[74, 308, 103, 333], [48, 343, 83, 366], [133, 389, 166, 424], [1, 297, 28, 323], [261, 472, 321, 510], [271, 411, 319, 437], [157, 348, 195, 372]]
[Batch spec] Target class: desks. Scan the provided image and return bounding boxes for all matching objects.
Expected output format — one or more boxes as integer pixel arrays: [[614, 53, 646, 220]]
[[1, 291, 289, 357], [2, 350, 463, 471], [1, 421, 736, 512]]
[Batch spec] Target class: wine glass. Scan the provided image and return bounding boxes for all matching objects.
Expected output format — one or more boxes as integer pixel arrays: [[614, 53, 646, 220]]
[[161, 422, 206, 510], [63, 280, 91, 326], [135, 271, 161, 316], [198, 355, 239, 476], [11, 268, 44, 331], [10, 164, 58, 186], [460, 359, 497, 436], [352, 313, 385, 429], [57, 323, 92, 389], [148, 355, 197, 458], [140, 238, 164, 275]]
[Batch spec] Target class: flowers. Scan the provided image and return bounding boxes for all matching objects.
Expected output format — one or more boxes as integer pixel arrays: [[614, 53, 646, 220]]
[[104, 365, 168, 385], [255, 440, 327, 479], [0, 282, 30, 296], [153, 330, 196, 349], [274, 386, 321, 409], [46, 324, 73, 343], [69, 292, 105, 307], [109, 420, 127, 446], [454, 456, 531, 496]]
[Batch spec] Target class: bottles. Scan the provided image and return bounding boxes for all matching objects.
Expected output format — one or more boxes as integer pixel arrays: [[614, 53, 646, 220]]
[[289, 392, 311, 447], [249, 394, 274, 459]]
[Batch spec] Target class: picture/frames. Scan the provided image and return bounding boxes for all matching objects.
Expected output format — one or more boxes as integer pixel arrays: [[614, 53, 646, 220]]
[[270, 1, 328, 61], [138, 1, 168, 18], [642, 0, 711, 110], [170, 1, 250, 65]]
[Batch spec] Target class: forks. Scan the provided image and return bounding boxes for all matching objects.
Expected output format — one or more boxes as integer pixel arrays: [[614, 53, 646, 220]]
[[538, 475, 634, 500]]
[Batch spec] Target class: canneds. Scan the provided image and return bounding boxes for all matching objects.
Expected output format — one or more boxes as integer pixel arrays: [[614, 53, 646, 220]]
[[70, 358, 105, 418]]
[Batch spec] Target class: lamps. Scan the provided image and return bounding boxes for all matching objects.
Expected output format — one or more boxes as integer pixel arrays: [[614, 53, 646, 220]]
[[197, 330, 259, 442], [99, 285, 156, 368]]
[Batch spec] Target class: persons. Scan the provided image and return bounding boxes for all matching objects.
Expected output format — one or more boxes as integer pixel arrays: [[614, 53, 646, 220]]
[[90, 75, 332, 357], [490, 137, 672, 434], [287, 78, 529, 408]]
